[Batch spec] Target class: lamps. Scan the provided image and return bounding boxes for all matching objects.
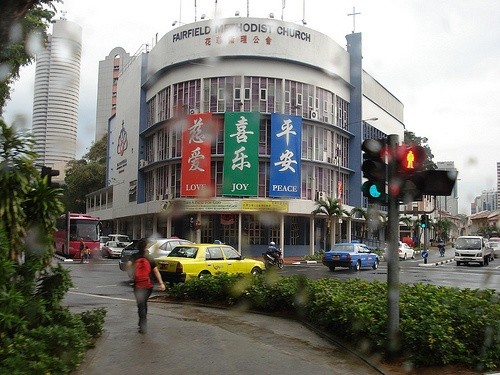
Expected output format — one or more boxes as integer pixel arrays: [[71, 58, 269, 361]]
[[235, 11, 240, 17], [302, 19, 307, 24], [269, 13, 274, 19], [171, 20, 187, 26], [200, 14, 209, 20]]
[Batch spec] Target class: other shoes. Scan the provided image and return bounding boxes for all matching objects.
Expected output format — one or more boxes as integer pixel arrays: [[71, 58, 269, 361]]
[[83, 261, 86, 263], [85, 261, 89, 263], [137, 325, 143, 333], [272, 261, 277, 265]]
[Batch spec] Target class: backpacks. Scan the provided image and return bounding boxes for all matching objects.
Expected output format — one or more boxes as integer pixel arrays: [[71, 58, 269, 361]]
[[133, 252, 153, 288]]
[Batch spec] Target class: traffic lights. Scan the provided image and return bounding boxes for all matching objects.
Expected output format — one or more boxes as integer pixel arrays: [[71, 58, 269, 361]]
[[420, 215, 427, 228], [402, 149, 424, 170], [47, 167, 60, 188], [361, 138, 386, 200]]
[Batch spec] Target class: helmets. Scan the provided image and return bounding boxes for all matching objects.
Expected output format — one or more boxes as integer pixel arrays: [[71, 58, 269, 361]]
[[441, 240, 443, 242], [270, 242, 275, 245]]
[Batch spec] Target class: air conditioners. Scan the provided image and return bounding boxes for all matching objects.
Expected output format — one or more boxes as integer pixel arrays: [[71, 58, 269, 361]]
[[309, 110, 317, 119], [189, 109, 199, 115], [156, 193, 173, 201], [317, 192, 326, 202]]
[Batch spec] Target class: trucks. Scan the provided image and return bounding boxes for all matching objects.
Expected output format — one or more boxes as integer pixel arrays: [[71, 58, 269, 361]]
[[451, 236, 492, 267]]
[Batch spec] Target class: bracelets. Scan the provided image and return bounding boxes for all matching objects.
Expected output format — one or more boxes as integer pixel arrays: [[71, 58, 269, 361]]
[[160, 283, 164, 285]]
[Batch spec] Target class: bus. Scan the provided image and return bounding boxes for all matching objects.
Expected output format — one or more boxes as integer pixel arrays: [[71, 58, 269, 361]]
[[51, 212, 104, 259]]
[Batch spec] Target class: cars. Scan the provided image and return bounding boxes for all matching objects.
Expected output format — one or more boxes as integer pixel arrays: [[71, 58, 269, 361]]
[[118, 237, 198, 278], [381, 242, 416, 261], [102, 241, 127, 259], [483, 237, 500, 261], [154, 239, 266, 284], [322, 242, 380, 271], [99, 233, 133, 250]]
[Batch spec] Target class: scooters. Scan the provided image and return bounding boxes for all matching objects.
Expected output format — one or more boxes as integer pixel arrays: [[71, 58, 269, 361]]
[[437, 244, 445, 257], [261, 248, 284, 269]]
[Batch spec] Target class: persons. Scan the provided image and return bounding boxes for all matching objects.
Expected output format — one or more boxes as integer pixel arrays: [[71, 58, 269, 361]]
[[127, 240, 166, 334], [267, 242, 282, 265], [70, 229, 89, 264], [438, 239, 446, 254]]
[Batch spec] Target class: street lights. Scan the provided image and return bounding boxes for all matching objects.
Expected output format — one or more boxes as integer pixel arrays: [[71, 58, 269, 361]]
[[336, 118, 379, 241]]
